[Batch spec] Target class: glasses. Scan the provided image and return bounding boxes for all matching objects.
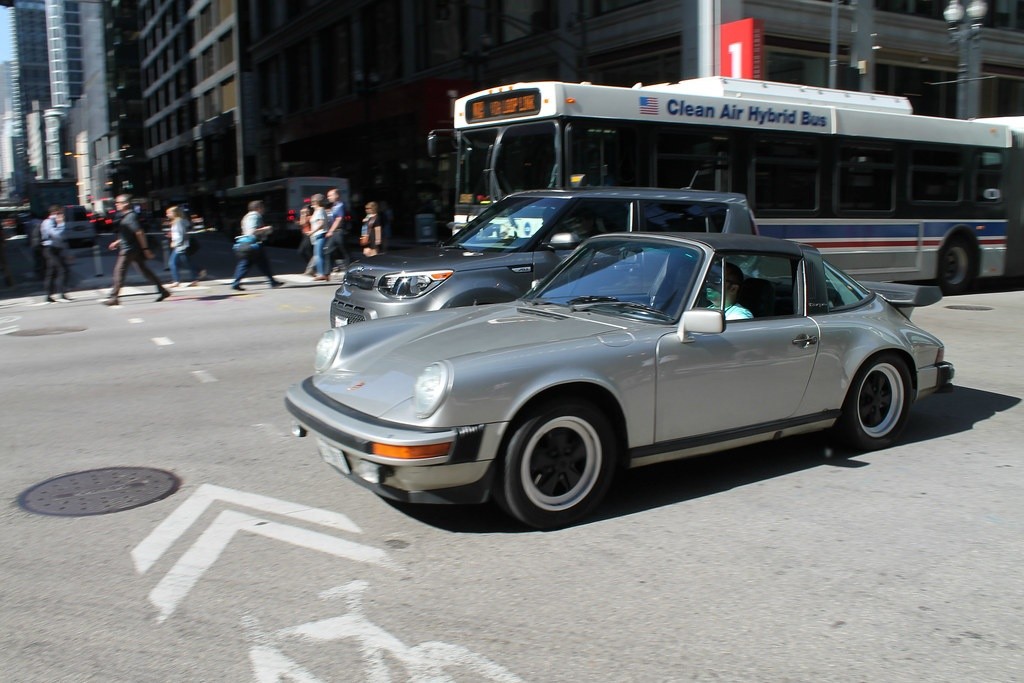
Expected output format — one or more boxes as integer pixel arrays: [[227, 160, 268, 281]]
[[113, 201, 127, 204], [706, 276, 737, 286]]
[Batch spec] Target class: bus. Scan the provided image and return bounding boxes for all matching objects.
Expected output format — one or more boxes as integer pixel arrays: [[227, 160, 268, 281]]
[[220, 176, 350, 246], [425, 75, 1024, 293]]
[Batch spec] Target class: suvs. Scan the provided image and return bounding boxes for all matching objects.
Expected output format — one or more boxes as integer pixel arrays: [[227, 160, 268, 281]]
[[326, 184, 762, 329]]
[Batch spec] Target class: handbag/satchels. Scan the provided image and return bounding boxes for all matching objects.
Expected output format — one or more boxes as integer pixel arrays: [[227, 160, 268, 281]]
[[360, 235, 371, 245], [231, 235, 259, 258]]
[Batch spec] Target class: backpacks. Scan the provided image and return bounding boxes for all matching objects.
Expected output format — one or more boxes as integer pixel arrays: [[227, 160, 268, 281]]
[[30, 224, 45, 259], [184, 236, 201, 255]]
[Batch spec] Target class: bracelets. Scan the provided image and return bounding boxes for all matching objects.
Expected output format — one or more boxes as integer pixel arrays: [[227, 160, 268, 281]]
[[142, 248, 150, 250]]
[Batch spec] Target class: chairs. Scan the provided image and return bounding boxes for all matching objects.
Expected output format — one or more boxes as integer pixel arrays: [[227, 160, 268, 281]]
[[745, 277, 777, 317]]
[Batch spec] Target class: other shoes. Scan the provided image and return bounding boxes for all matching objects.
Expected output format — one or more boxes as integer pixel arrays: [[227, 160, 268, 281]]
[[168, 282, 178, 289], [103, 299, 118, 306], [315, 275, 329, 281], [63, 294, 73, 301], [187, 281, 199, 288], [157, 291, 171, 303], [46, 298, 55, 302], [272, 281, 284, 287], [233, 286, 246, 291]]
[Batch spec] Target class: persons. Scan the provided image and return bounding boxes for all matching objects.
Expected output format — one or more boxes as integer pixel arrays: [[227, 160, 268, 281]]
[[100, 193, 171, 306], [297, 188, 353, 281], [230, 200, 285, 290], [166, 205, 207, 287], [361, 201, 382, 258], [705, 261, 754, 320], [30, 205, 74, 303]]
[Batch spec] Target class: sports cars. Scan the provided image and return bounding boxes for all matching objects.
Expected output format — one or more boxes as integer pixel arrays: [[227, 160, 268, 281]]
[[284, 225, 959, 530]]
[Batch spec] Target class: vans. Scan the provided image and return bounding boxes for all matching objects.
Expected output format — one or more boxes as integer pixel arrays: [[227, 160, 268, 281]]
[[63, 203, 99, 249]]
